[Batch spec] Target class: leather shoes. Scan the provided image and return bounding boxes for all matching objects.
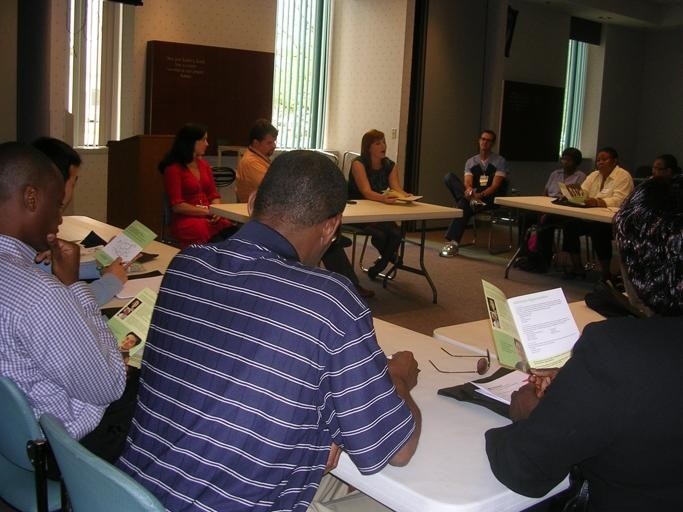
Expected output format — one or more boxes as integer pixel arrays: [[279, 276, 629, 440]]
[[386, 249, 398, 263], [368, 254, 389, 280], [334, 234, 352, 248], [355, 284, 375, 297]]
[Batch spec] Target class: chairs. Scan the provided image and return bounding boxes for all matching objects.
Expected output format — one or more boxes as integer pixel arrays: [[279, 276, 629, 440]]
[[160, 191, 179, 246], [511, 177, 650, 269], [458, 174, 512, 255], [340, 151, 405, 279], [39, 412, 164, 512], [0, 373, 71, 512], [217, 145, 247, 205]]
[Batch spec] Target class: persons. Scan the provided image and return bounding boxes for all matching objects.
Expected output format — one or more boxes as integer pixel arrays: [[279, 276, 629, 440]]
[[0, 142, 137, 482], [347, 129, 415, 278], [29, 135, 133, 309], [158, 123, 239, 248], [512, 147, 586, 272], [130, 299, 141, 310], [649, 154, 678, 178], [108, 150, 420, 510], [490, 311, 501, 329], [485, 179, 682, 511], [117, 332, 141, 363], [560, 147, 634, 281], [514, 338, 530, 373], [117, 307, 132, 319], [487, 298, 497, 313], [236, 116, 375, 298], [437, 127, 507, 260]]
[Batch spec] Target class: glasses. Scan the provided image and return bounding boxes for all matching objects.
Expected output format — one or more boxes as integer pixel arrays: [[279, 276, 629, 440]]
[[428, 348, 490, 375]]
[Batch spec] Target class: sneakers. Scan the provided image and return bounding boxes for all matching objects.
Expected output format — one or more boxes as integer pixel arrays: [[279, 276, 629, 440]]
[[441, 240, 459, 257], [470, 199, 487, 211]]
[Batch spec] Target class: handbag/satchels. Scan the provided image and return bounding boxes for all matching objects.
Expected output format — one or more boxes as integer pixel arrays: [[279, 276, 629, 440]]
[[521, 223, 553, 274]]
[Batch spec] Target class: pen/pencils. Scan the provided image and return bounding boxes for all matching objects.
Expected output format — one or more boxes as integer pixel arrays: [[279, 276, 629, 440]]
[[523, 373, 553, 381], [123, 254, 141, 269]]
